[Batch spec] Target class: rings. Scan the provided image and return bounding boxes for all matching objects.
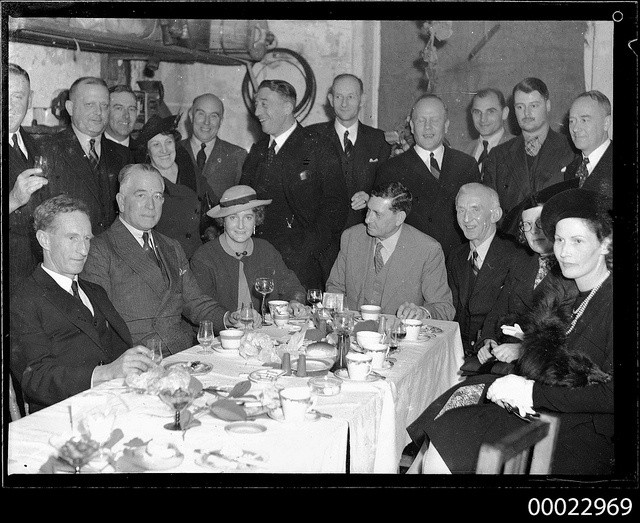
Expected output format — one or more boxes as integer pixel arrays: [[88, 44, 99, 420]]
[[363, 199, 367, 203]]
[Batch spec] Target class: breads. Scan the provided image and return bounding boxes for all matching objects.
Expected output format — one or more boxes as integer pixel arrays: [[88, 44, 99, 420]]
[[305, 342, 337, 357]]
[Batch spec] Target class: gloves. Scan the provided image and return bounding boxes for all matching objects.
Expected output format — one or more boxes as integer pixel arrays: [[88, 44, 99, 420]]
[[486, 374, 536, 408]]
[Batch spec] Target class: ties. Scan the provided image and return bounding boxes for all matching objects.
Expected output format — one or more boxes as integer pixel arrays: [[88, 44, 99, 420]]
[[12, 134, 27, 162], [478, 141, 489, 173], [373, 242, 385, 275], [344, 131, 354, 161], [525, 137, 541, 156], [71, 281, 92, 317], [264, 140, 277, 189], [142, 232, 159, 267], [471, 251, 479, 288], [430, 153, 441, 179], [575, 159, 590, 188], [197, 143, 206, 173], [89, 139, 100, 175]]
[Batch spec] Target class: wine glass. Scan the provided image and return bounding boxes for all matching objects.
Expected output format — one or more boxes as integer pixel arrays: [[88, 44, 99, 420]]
[[240, 303, 254, 332], [255, 277, 274, 325], [33, 156, 49, 177], [158, 387, 195, 430], [196, 320, 214, 355], [145, 338, 163, 365], [307, 289, 322, 314], [392, 316, 406, 352], [325, 294, 343, 330]]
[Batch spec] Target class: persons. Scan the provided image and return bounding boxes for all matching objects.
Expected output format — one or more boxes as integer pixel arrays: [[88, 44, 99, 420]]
[[374, 94, 483, 261], [483, 76, 582, 248], [189, 184, 308, 346], [453, 88, 517, 182], [404, 175, 581, 474], [422, 187, 614, 475], [180, 93, 247, 200], [129, 108, 224, 261], [325, 180, 456, 321], [303, 73, 391, 291], [79, 163, 263, 358], [10, 194, 163, 415], [102, 84, 138, 147], [37, 78, 135, 234], [239, 79, 349, 305], [564, 90, 613, 196], [8, 62, 48, 302], [446, 182, 538, 356]]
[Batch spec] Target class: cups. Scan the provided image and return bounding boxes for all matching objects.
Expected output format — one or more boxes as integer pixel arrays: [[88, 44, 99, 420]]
[[220, 329, 244, 349], [401, 319, 422, 341], [268, 300, 289, 319], [345, 354, 372, 380], [360, 304, 380, 321], [280, 387, 311, 422], [356, 330, 382, 348], [334, 312, 355, 336], [274, 314, 289, 326], [363, 344, 391, 368]]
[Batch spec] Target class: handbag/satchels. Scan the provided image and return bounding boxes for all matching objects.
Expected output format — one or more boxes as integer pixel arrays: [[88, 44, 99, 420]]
[[460, 355, 516, 376]]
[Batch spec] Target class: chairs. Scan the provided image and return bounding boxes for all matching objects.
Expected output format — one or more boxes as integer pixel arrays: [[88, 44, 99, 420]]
[[9, 374, 29, 422], [475, 413, 561, 474]]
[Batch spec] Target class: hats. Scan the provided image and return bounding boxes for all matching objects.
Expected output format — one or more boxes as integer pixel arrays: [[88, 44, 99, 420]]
[[502, 176, 579, 234], [140, 109, 184, 144], [206, 185, 272, 218], [541, 188, 614, 242]]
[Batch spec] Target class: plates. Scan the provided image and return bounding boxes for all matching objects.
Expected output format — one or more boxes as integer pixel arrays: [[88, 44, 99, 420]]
[[362, 360, 394, 370], [268, 407, 322, 424], [334, 368, 380, 383], [353, 316, 365, 322], [401, 335, 431, 343], [211, 342, 239, 353], [220, 420, 269, 436], [164, 361, 214, 376]]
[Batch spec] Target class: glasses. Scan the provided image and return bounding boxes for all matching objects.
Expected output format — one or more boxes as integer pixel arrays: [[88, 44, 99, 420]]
[[520, 218, 544, 232]]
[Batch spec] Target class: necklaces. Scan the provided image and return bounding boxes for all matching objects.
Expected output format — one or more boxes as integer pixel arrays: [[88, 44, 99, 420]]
[[565, 269, 610, 335]]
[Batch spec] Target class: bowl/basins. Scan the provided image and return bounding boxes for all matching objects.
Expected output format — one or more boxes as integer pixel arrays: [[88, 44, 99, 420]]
[[291, 359, 334, 372], [248, 369, 278, 382]]
